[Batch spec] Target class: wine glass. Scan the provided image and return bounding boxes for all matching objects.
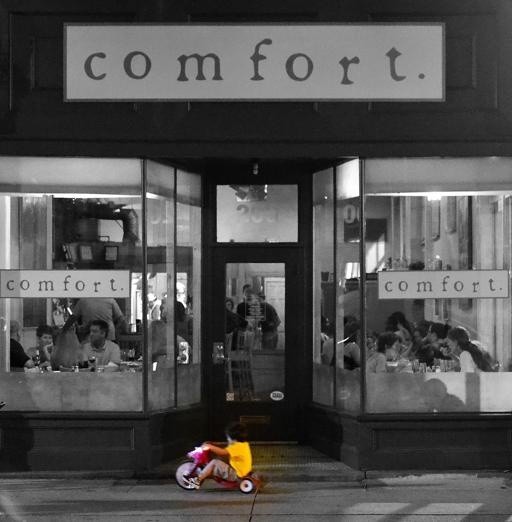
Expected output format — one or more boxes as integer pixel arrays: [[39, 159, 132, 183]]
[[88, 355, 96, 369]]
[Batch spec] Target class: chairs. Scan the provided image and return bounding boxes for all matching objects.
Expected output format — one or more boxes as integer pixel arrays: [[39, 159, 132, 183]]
[[229, 329, 256, 400]]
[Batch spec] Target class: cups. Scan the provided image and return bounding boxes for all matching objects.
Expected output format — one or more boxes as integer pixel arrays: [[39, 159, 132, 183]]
[[96, 358, 104, 373], [410, 359, 456, 373]]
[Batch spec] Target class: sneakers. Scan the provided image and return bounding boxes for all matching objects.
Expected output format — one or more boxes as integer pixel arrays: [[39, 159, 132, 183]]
[[182, 475, 201, 489]]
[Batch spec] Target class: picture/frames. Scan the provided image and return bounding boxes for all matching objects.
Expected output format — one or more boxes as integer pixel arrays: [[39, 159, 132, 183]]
[[79, 244, 119, 263]]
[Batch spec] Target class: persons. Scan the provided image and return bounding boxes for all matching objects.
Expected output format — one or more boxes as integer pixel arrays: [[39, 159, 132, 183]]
[[226, 283, 281, 352], [319, 311, 500, 374], [182, 422, 253, 490], [5, 292, 192, 373]]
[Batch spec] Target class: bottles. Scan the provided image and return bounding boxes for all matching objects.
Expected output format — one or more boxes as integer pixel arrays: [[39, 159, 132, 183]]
[[72, 362, 79, 372]]
